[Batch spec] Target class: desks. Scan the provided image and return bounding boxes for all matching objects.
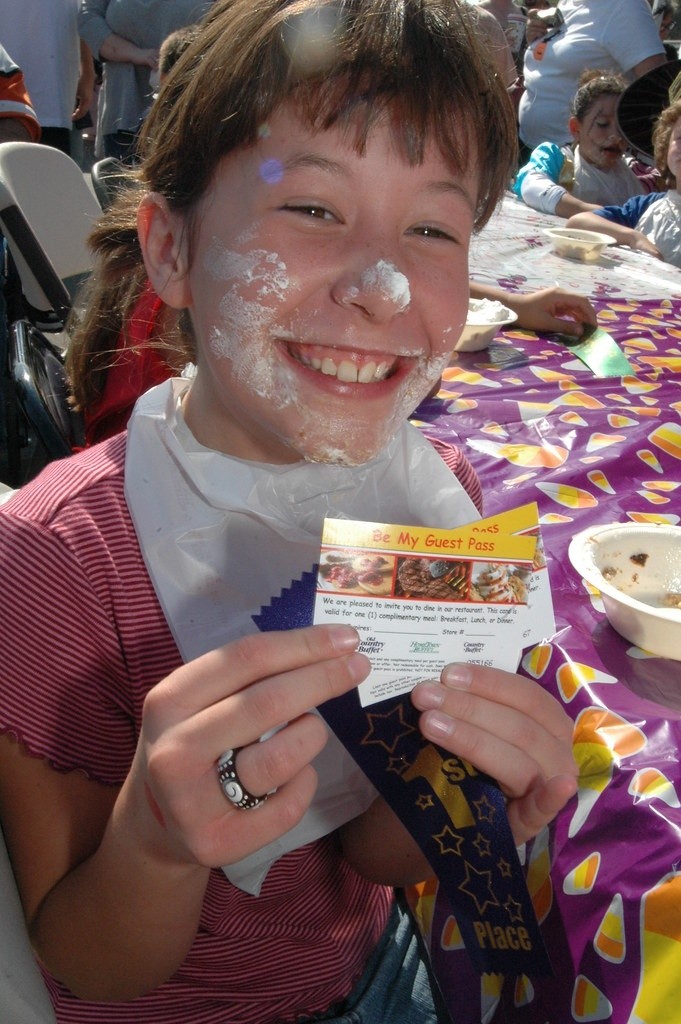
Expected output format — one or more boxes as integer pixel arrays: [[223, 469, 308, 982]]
[[406, 196, 681, 1024]]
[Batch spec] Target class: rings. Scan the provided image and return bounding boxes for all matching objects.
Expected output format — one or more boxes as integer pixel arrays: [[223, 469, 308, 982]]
[[218, 748, 267, 811]]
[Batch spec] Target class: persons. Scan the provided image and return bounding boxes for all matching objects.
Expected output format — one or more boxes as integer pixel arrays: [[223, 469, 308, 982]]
[[67, 266, 193, 442], [0, 0, 96, 155], [465, 0, 681, 267], [79, 0, 217, 170], [0, 0, 580, 1023]]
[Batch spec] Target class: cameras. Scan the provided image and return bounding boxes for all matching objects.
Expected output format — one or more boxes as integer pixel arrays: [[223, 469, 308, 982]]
[[536, 8, 564, 28]]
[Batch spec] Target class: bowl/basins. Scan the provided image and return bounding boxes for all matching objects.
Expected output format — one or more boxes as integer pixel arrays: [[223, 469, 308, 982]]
[[543, 227, 617, 260], [566, 521, 681, 662], [455, 298, 518, 350]]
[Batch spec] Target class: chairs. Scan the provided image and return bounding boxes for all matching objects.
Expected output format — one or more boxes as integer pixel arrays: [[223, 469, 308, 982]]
[[0, 141, 103, 337]]
